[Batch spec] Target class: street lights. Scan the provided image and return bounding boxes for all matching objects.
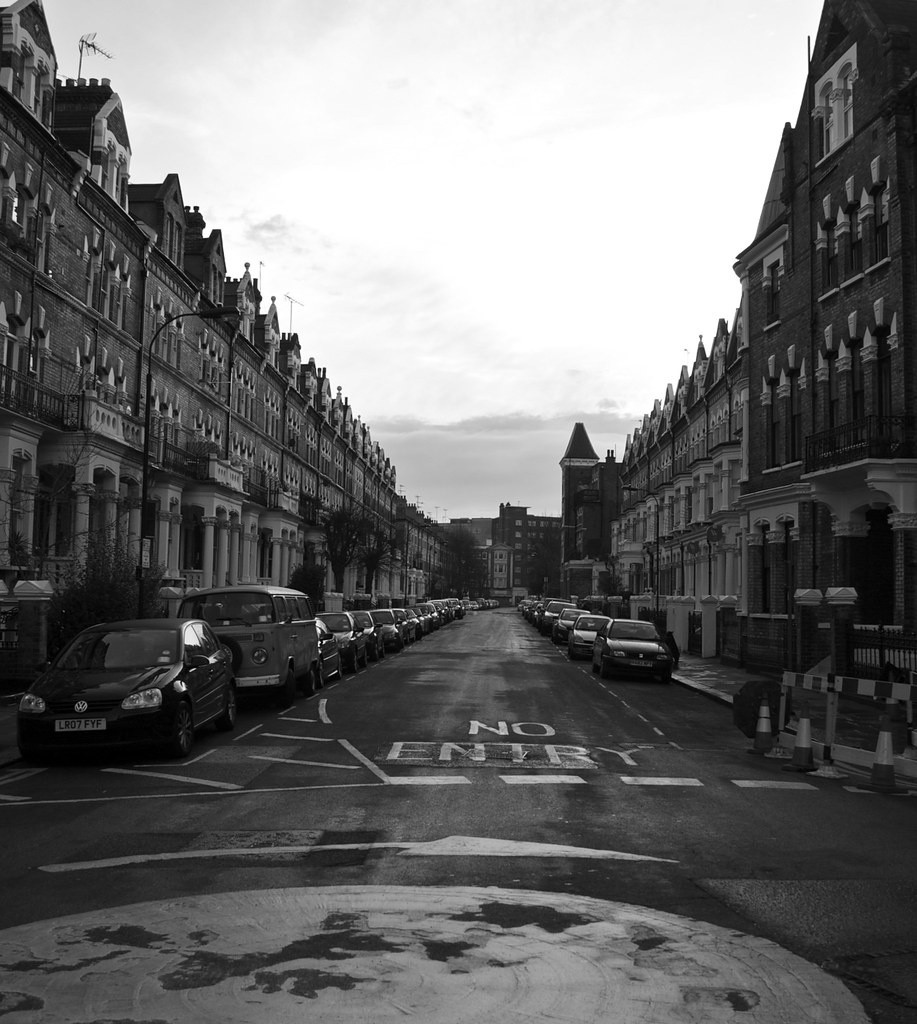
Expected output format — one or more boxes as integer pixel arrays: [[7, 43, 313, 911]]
[[621, 485, 661, 622], [134, 302, 242, 617]]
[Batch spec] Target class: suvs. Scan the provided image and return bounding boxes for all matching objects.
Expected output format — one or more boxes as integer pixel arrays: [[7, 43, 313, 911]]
[[315, 611, 368, 673], [539, 601, 578, 637]]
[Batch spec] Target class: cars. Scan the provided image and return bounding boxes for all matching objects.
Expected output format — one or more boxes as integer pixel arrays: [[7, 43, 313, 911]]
[[567, 614, 612, 660], [391, 596, 501, 647], [552, 608, 591, 646], [370, 608, 405, 654], [15, 617, 240, 761], [313, 616, 343, 688], [517, 597, 571, 633], [592, 618, 673, 685], [349, 611, 386, 661]]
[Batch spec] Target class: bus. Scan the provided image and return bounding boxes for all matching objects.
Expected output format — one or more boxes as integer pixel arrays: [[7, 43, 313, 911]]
[[176, 586, 321, 710]]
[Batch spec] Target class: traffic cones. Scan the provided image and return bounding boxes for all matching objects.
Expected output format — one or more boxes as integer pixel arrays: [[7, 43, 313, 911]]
[[780, 702, 820, 773], [855, 714, 911, 795], [745, 696, 777, 755]]
